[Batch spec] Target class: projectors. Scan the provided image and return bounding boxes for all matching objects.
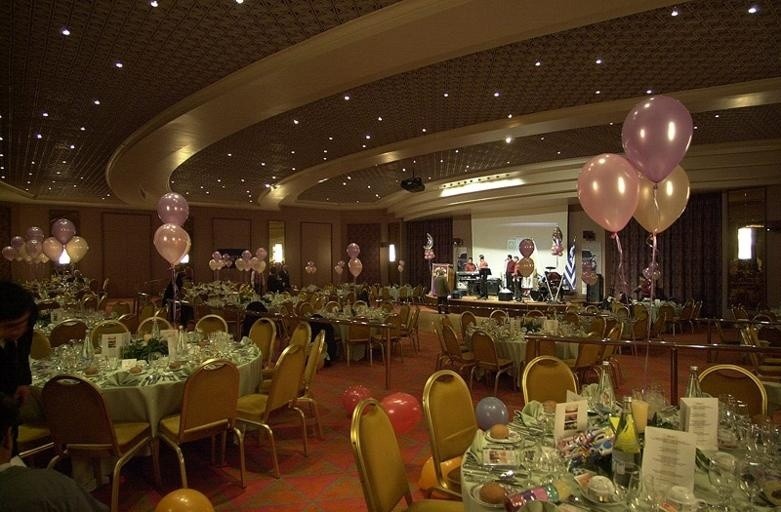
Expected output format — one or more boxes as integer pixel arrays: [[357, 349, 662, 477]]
[[399, 176, 425, 194]]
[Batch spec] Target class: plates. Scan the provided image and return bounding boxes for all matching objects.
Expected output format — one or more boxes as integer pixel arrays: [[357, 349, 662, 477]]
[[468, 479, 518, 508], [166, 365, 185, 372], [83, 372, 104, 376], [580, 475, 618, 506], [486, 429, 523, 443], [128, 369, 148, 376]]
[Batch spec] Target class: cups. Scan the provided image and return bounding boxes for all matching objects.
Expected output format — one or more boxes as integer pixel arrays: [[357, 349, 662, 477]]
[[585, 473, 617, 501], [709, 452, 742, 512], [748, 415, 773, 484], [770, 425, 781, 471], [665, 485, 709, 512], [739, 456, 766, 512], [727, 399, 750, 449], [714, 392, 737, 433], [628, 470, 657, 512], [634, 386, 666, 420], [614, 461, 643, 503]]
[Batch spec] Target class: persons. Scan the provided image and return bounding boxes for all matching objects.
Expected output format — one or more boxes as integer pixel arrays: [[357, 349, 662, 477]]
[[466, 257, 478, 295], [435, 269, 452, 314], [0, 283, 36, 463], [0, 398, 112, 512], [476, 255, 489, 301], [510, 257, 523, 301], [506, 256, 514, 289]]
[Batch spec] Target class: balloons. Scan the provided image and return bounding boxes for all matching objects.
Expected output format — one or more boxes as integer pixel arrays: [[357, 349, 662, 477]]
[[10, 236, 23, 247], [580, 261, 600, 286], [622, 98, 693, 184], [476, 396, 508, 432], [157, 192, 188, 224], [25, 240, 41, 263], [631, 163, 691, 235], [348, 259, 362, 277], [305, 261, 318, 274], [2, 246, 17, 260], [377, 392, 422, 433], [517, 239, 533, 277], [423, 248, 435, 260], [578, 154, 639, 234], [397, 259, 406, 272], [643, 260, 662, 282], [26, 226, 44, 245], [346, 244, 361, 257], [335, 261, 345, 276], [152, 490, 213, 512], [42, 238, 63, 263], [341, 385, 373, 417], [210, 247, 267, 272], [551, 240, 563, 256], [51, 219, 75, 245], [66, 236, 86, 260], [156, 225, 186, 264]]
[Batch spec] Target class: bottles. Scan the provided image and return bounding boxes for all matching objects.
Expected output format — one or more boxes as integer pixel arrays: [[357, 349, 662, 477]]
[[686, 366, 703, 398], [177, 326, 187, 356], [596, 362, 618, 415], [151, 319, 161, 341], [613, 396, 642, 487], [83, 329, 96, 361]]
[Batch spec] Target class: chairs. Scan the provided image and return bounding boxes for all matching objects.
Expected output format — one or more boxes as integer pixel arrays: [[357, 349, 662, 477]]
[[566, 305, 578, 312], [658, 303, 677, 337], [395, 310, 419, 353], [441, 316, 466, 345], [374, 313, 404, 366], [311, 291, 318, 302], [314, 296, 322, 308], [89, 279, 98, 291], [398, 304, 411, 327], [281, 291, 290, 298], [412, 287, 421, 305], [586, 304, 598, 313], [461, 312, 476, 337], [749, 315, 771, 327], [298, 292, 307, 299], [295, 301, 303, 315], [282, 299, 294, 316], [742, 304, 751, 316], [430, 320, 449, 372], [151, 309, 168, 322], [49, 317, 89, 348], [400, 287, 408, 304], [691, 299, 703, 320], [565, 312, 578, 324], [352, 300, 366, 312], [527, 310, 544, 319], [423, 369, 477, 499], [602, 327, 619, 390], [137, 317, 172, 335], [42, 373, 159, 511], [633, 303, 648, 320], [563, 332, 603, 397], [118, 313, 140, 333], [370, 286, 377, 298], [266, 291, 274, 298], [259, 329, 326, 444], [192, 295, 204, 323], [224, 302, 245, 341], [382, 287, 393, 304], [731, 305, 745, 328], [99, 278, 109, 296], [346, 292, 354, 303], [521, 356, 579, 410], [679, 298, 694, 336], [624, 318, 647, 359], [593, 316, 607, 340], [84, 296, 97, 311], [617, 307, 629, 321], [747, 327, 781, 375], [755, 302, 770, 313], [261, 323, 312, 379], [713, 317, 741, 364], [468, 331, 514, 394], [519, 336, 556, 385], [299, 302, 312, 315], [247, 318, 277, 370], [613, 321, 625, 384], [97, 294, 109, 310], [326, 301, 341, 314], [330, 321, 345, 359], [546, 305, 557, 312], [154, 357, 247, 495], [346, 314, 375, 367], [698, 365, 767, 421], [221, 342, 309, 479], [15, 422, 55, 458], [194, 315, 227, 336], [90, 320, 129, 346], [739, 328, 781, 390], [441, 325, 474, 383], [136, 303, 154, 320], [351, 396, 466, 511], [401, 306, 420, 351], [490, 309, 509, 326], [752, 328, 781, 366], [110, 301, 131, 314]]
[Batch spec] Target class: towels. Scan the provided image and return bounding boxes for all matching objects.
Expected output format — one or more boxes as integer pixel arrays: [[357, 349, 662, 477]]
[[240, 336, 252, 346], [513, 399, 544, 426], [31, 358, 56, 373], [108, 372, 137, 386], [580, 383, 599, 410], [515, 500, 561, 512], [471, 430, 487, 463]]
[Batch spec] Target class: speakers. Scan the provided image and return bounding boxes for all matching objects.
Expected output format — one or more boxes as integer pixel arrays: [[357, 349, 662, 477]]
[[586, 274, 605, 302]]
